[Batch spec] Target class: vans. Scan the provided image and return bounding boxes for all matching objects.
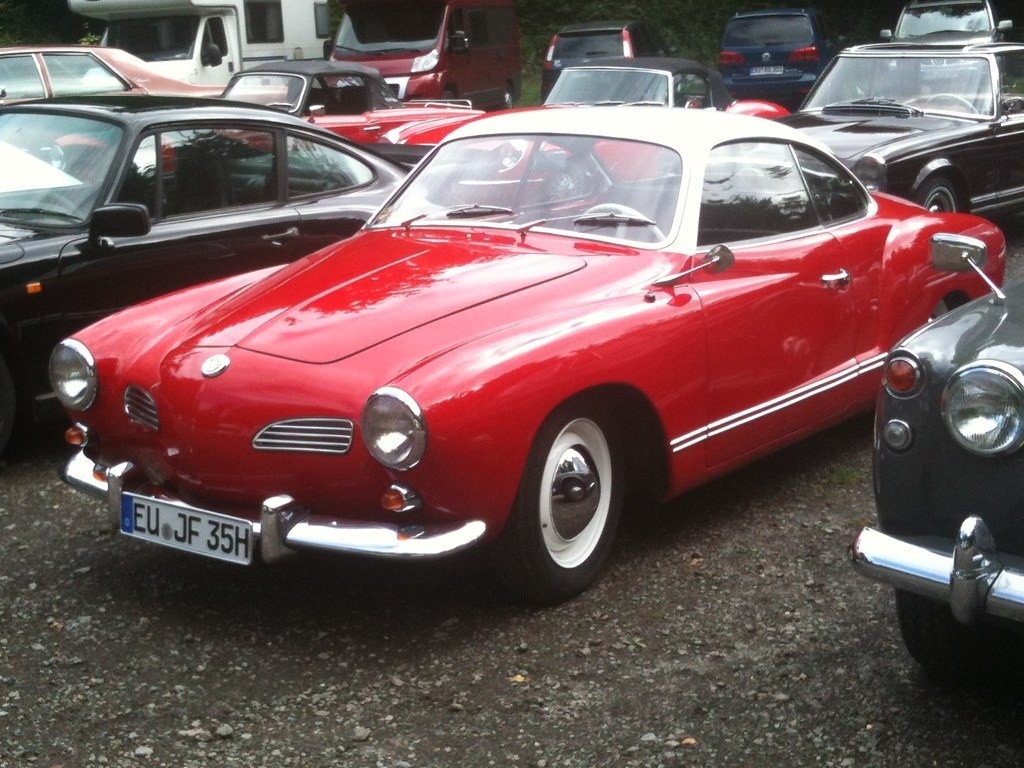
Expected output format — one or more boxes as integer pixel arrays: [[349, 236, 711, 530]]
[[327, 0, 522, 112]]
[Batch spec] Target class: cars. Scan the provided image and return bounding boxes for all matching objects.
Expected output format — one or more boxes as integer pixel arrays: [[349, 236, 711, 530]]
[[49, 107, 1005, 609], [1, 98, 414, 465], [847, 231, 1024, 680], [748, 43, 1023, 213], [541, 19, 677, 102], [0, 46, 287, 147]]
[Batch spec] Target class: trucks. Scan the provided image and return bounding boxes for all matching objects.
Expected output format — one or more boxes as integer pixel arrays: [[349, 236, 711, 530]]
[[67, 1, 332, 87]]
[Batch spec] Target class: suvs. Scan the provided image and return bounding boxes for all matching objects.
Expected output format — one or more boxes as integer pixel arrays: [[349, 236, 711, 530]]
[[718, 7, 846, 101], [879, 0, 1013, 92]]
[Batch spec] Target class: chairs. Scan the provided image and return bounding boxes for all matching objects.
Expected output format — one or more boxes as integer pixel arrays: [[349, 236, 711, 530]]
[[145, 143, 229, 218], [63, 145, 149, 209]]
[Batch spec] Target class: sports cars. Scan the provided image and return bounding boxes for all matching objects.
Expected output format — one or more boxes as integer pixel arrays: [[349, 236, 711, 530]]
[[50, 60, 486, 175], [376, 56, 793, 219]]
